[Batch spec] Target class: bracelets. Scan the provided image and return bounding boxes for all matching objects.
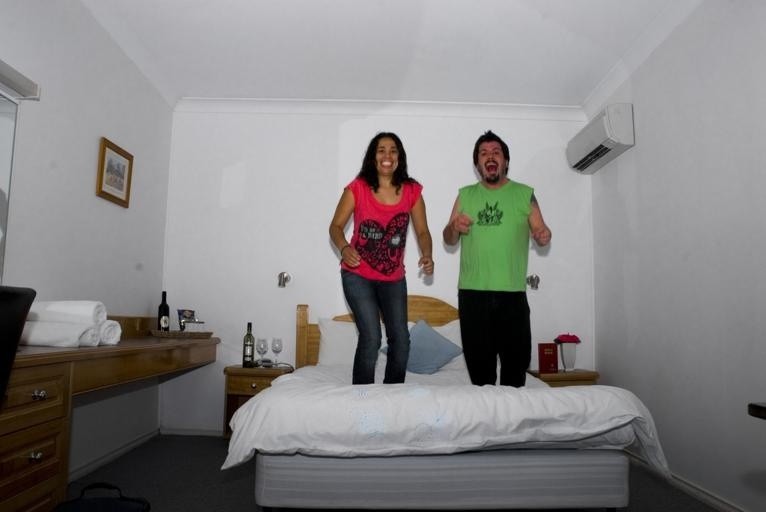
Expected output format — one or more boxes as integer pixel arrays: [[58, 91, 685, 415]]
[[340, 244, 350, 256]]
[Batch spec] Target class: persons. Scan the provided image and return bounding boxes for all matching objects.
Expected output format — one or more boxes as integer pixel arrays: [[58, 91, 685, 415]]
[[327, 130, 435, 384], [442, 128, 553, 385]]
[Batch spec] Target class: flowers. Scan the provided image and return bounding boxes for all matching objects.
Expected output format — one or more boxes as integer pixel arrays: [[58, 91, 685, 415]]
[[556, 332, 582, 345]]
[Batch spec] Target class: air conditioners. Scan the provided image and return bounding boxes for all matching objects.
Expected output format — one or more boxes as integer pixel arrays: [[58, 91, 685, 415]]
[[565, 102, 635, 176]]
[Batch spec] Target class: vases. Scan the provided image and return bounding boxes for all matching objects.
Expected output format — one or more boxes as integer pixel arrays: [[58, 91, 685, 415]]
[[558, 342, 577, 372]]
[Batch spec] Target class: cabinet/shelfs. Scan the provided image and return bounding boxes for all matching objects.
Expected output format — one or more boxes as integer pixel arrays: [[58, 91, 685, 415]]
[[1, 348, 73, 512]]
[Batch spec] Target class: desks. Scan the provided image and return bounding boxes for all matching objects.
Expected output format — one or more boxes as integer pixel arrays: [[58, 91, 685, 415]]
[[71, 334, 221, 397]]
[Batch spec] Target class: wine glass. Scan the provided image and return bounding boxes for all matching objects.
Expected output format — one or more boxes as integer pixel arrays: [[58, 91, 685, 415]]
[[272, 338, 284, 369], [256, 339, 268, 369]]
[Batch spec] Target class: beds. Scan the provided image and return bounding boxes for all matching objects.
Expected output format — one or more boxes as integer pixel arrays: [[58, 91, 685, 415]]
[[220, 295, 672, 510]]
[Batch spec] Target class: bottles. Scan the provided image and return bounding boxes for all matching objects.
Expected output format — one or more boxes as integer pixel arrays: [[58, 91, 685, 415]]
[[243, 322, 255, 368], [157, 291, 169, 332]]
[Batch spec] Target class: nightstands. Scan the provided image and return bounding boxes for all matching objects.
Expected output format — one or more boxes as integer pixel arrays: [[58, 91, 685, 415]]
[[221, 364, 292, 439], [526, 370, 601, 386]]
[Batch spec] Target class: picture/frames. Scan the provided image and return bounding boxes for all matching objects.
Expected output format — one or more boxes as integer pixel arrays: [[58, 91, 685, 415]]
[[96, 136, 137, 207]]
